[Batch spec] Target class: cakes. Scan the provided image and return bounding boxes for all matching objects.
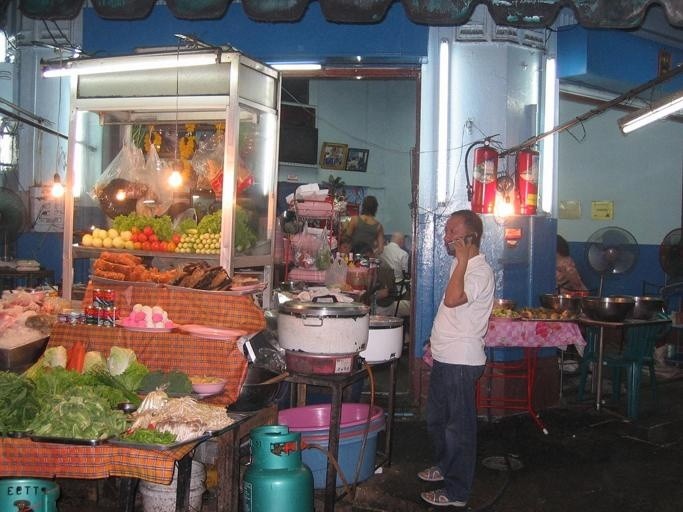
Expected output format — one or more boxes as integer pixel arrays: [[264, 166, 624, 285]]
[[122, 303, 175, 330]]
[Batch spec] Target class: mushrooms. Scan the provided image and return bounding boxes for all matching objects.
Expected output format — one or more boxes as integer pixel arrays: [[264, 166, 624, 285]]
[[128, 390, 236, 441]]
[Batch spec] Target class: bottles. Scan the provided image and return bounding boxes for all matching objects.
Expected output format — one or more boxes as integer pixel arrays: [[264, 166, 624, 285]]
[[334, 252, 364, 268], [670, 311, 683, 326]]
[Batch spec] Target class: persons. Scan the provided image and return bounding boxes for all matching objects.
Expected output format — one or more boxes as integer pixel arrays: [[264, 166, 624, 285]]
[[339, 196, 410, 317], [417, 210, 496, 507]]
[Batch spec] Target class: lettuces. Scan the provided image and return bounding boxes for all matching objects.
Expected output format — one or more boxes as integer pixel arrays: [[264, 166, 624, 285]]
[[110, 363, 150, 398]]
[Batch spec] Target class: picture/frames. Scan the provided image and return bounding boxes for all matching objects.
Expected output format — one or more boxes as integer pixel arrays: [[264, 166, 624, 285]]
[[344, 146, 369, 172], [657, 49, 674, 84], [317, 142, 347, 172]]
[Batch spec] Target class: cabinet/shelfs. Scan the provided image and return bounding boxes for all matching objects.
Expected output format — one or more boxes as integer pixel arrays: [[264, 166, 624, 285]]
[[290, 198, 343, 266], [36, 51, 281, 316]]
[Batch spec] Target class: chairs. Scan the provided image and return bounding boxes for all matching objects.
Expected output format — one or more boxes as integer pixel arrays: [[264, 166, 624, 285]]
[[576, 305, 672, 424]]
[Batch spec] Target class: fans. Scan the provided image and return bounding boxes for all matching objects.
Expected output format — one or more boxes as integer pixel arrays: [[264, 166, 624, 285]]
[[582, 223, 641, 296], [657, 227, 682, 310], [0, 186, 28, 262]]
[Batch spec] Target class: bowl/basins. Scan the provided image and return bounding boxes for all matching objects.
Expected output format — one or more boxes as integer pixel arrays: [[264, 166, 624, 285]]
[[271, 402, 385, 489], [189, 377, 226, 394], [492, 298, 516, 309], [538, 292, 665, 322], [224, 366, 288, 412]]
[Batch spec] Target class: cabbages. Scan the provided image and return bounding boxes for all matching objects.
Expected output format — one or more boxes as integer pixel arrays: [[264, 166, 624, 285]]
[[106, 345, 136, 377], [80, 350, 101, 374], [44, 345, 67, 369]]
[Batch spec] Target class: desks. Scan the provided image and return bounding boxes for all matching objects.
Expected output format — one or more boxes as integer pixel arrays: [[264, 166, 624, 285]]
[[87, 403, 278, 510], [0, 267, 54, 296], [285, 261, 376, 297], [422, 316, 587, 435]]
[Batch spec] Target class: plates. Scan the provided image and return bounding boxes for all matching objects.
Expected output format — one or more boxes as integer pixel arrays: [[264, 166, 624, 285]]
[[177, 323, 248, 341], [114, 320, 179, 333], [230, 281, 267, 291]]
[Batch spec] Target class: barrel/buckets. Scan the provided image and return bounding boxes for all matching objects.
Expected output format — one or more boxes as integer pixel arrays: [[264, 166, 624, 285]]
[[194, 439, 220, 503], [138, 460, 209, 512]]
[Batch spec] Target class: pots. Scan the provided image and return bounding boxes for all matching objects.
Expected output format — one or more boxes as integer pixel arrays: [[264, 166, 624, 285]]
[[275, 293, 404, 365]]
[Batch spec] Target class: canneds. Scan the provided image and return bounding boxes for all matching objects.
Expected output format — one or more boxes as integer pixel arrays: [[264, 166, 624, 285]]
[[58, 288, 120, 327]]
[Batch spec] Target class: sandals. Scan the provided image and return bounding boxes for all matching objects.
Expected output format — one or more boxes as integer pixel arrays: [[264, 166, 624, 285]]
[[418, 464, 444, 481], [420, 488, 466, 507]]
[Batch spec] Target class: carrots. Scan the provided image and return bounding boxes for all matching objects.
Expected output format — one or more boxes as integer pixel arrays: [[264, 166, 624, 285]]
[[67, 341, 89, 372]]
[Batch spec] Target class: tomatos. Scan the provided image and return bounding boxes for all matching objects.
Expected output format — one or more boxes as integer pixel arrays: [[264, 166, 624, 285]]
[[130, 225, 180, 252]]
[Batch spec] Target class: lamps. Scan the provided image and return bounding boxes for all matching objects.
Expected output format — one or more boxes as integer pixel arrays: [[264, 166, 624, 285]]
[[492, 151, 515, 217], [167, 34, 203, 188], [616, 78, 682, 136], [40, 56, 91, 79], [48, 43, 68, 202]]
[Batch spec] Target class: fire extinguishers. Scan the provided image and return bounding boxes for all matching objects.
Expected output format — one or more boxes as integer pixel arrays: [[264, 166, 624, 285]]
[[465, 134, 502, 214], [514, 136, 539, 215]]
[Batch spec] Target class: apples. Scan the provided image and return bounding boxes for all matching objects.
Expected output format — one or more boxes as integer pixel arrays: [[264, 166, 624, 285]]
[[82, 228, 133, 250]]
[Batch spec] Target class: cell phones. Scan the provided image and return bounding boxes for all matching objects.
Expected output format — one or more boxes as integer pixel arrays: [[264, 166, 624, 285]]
[[447, 233, 475, 251]]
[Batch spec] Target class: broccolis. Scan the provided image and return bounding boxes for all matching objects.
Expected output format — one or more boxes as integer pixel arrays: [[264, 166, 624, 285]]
[[160, 368, 193, 398], [135, 369, 167, 396]]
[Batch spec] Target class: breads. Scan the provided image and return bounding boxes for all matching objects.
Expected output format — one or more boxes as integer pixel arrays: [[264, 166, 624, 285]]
[[94, 251, 260, 292]]
